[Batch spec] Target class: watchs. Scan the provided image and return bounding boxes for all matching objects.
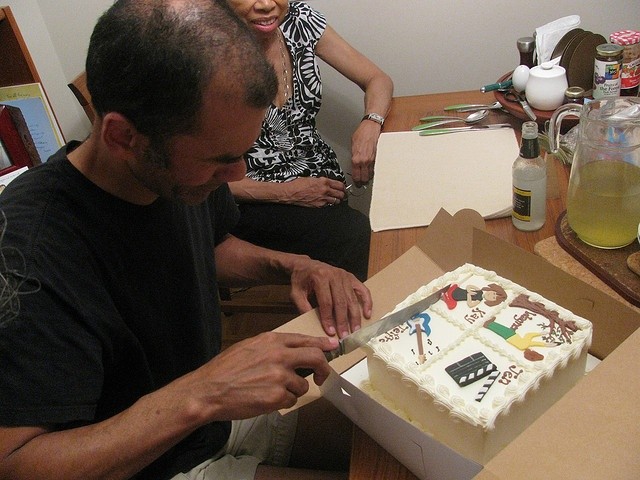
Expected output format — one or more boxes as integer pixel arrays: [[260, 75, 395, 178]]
[[361, 113, 384, 131]]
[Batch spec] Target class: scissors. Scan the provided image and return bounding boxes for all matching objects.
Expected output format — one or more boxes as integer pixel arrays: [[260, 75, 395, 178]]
[[504, 90, 537, 121]]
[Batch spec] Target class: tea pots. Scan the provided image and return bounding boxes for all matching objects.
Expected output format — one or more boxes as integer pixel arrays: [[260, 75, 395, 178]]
[[548, 95, 640, 251]]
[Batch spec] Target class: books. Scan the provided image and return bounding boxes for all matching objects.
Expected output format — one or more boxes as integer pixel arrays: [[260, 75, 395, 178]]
[[0, 83, 66, 147]]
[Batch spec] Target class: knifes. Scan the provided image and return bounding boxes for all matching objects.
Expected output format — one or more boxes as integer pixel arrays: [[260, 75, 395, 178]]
[[419, 123, 513, 136], [294, 283, 452, 379]]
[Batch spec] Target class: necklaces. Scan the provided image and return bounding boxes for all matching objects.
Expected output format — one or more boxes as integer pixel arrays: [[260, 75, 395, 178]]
[[275, 31, 290, 104]]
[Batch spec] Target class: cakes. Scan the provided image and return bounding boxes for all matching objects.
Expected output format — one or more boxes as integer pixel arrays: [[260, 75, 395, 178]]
[[360, 262, 593, 466]]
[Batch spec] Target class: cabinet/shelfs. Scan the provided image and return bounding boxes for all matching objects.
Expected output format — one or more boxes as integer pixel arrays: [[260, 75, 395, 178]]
[[1, 5, 67, 143]]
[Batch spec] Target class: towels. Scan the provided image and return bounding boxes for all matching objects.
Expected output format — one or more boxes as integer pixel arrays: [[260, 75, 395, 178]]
[[369, 126, 520, 233]]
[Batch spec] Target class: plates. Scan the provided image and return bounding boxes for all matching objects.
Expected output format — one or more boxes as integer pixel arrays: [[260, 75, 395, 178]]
[[558, 31, 595, 81], [567, 34, 608, 92], [550, 27, 584, 61]]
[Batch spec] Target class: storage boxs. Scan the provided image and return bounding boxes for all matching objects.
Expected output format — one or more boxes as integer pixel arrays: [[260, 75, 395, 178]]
[[271, 207, 640, 479]]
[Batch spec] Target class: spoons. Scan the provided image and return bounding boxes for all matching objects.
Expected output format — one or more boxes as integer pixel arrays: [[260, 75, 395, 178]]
[[419, 109, 490, 123], [411, 117, 487, 131], [456, 101, 503, 114]]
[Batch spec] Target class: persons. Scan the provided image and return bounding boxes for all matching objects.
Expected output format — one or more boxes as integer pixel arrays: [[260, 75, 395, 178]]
[[1, 0, 373, 479], [218, 0, 393, 281]]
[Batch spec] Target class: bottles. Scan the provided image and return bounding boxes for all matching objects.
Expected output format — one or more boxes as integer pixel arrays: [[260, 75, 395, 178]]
[[511, 120, 547, 232], [563, 86, 585, 104], [517, 36, 535, 69]]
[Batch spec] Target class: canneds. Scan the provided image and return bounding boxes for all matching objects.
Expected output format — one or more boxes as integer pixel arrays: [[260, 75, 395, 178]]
[[593, 43, 623, 100], [608, 29, 640, 95], [564, 87, 585, 105]]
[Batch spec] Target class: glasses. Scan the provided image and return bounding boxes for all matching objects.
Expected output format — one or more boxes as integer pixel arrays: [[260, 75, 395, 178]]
[[346, 172, 367, 201]]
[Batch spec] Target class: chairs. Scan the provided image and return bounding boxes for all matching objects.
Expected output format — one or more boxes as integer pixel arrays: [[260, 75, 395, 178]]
[[69, 71, 231, 301]]
